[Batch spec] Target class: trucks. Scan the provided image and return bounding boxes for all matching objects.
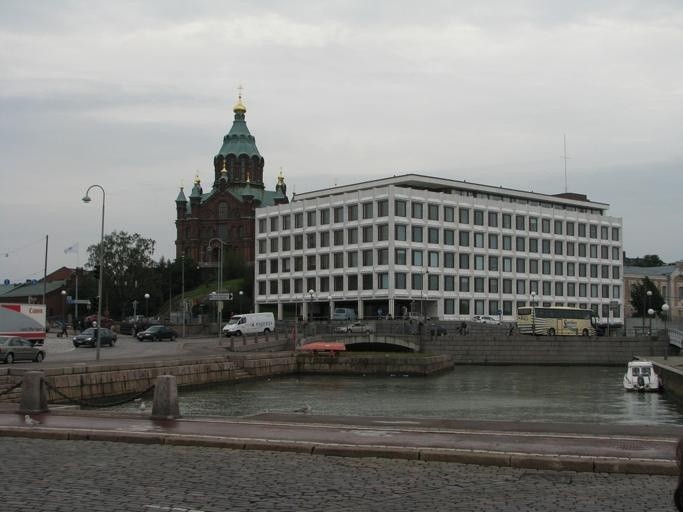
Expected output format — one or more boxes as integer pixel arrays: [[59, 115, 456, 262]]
[[221, 312, 276, 336]]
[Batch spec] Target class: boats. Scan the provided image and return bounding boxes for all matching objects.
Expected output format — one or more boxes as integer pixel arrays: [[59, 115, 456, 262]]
[[621, 358, 663, 392]]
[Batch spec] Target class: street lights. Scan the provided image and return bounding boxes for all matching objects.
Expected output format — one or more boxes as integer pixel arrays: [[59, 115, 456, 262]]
[[647, 291, 654, 307], [143, 292, 151, 318], [530, 288, 536, 336], [307, 288, 314, 324], [179, 249, 189, 340], [647, 307, 655, 359], [661, 302, 674, 361], [80, 182, 108, 359], [327, 295, 332, 320], [419, 264, 429, 316], [206, 236, 225, 346], [237, 289, 245, 314], [60, 290, 67, 324]]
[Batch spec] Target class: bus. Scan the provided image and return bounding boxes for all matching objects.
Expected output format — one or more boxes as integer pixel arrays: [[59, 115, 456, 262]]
[[515, 305, 600, 338]]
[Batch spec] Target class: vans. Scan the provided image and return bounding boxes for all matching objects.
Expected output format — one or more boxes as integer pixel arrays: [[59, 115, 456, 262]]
[[332, 307, 357, 320]]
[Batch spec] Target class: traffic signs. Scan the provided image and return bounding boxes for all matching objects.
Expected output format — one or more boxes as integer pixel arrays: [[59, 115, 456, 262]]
[[207, 291, 233, 300]]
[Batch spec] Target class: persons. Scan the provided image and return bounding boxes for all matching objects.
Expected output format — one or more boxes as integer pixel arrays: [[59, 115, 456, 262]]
[[509, 324, 513, 335], [459, 320, 466, 335], [62, 319, 68, 337]]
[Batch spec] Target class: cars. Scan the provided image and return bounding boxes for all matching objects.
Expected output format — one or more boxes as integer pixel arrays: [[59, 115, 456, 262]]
[[72, 312, 148, 348], [471, 314, 501, 326], [0, 335, 47, 365], [136, 324, 180, 344], [333, 321, 373, 335], [430, 323, 450, 336]]
